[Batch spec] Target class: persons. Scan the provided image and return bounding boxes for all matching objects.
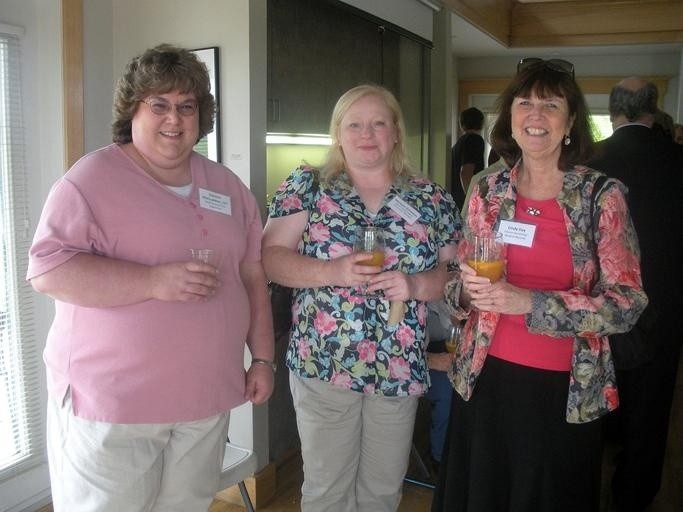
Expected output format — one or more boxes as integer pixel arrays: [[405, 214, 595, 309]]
[[446, 106, 486, 216], [22, 43, 280, 511], [574, 75, 682, 511], [651, 110, 675, 142], [438, 54, 651, 512], [254, 81, 465, 512], [669, 123, 682, 147]]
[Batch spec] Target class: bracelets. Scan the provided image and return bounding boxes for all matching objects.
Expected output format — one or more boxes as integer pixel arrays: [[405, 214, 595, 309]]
[[249, 358, 278, 374]]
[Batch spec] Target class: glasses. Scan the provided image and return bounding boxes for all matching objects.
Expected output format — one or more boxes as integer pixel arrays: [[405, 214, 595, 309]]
[[516, 57, 575, 81], [139, 96, 200, 117]]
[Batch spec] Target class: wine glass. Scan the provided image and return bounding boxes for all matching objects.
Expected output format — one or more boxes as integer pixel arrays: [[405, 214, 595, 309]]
[[352, 227, 386, 300], [466, 229, 506, 311]]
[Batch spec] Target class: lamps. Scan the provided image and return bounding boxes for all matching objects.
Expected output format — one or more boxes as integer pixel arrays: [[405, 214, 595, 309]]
[[266, 133, 336, 147]]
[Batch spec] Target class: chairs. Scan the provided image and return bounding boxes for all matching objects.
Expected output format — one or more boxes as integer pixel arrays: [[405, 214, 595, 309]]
[[218, 433, 258, 512]]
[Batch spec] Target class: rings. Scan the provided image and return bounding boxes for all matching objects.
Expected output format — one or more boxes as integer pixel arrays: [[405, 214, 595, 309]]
[[489, 297, 494, 304]]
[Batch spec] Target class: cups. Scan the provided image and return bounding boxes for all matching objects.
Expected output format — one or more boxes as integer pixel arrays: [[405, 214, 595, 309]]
[[184, 247, 222, 300], [446, 325, 464, 354]]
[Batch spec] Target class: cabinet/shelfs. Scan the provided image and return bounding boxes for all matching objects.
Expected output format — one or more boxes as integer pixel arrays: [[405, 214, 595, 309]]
[[267, 0, 401, 135]]
